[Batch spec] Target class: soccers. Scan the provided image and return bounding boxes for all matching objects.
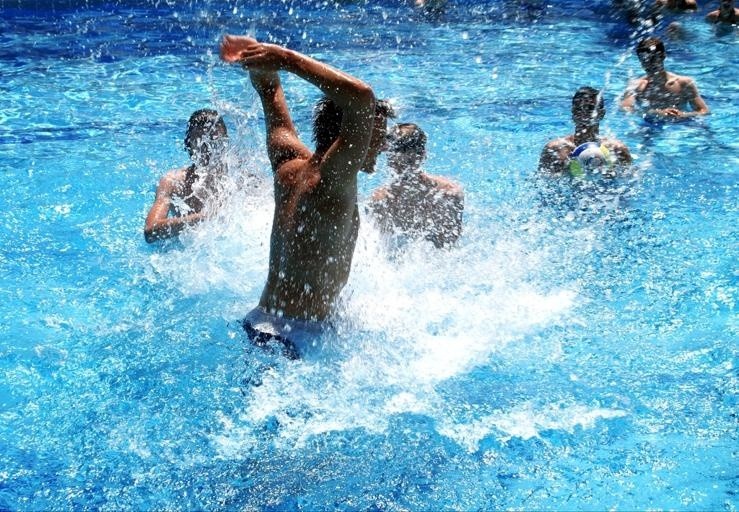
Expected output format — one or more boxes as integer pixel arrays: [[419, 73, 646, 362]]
[[570, 142, 611, 182]]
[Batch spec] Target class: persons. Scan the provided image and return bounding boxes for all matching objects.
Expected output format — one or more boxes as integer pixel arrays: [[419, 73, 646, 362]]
[[656, 0, 697, 13], [619, 38, 710, 122], [538, 86, 631, 169], [371, 122, 464, 248], [145, 109, 238, 243], [705, 0, 739, 24], [219, 33, 375, 359]]
